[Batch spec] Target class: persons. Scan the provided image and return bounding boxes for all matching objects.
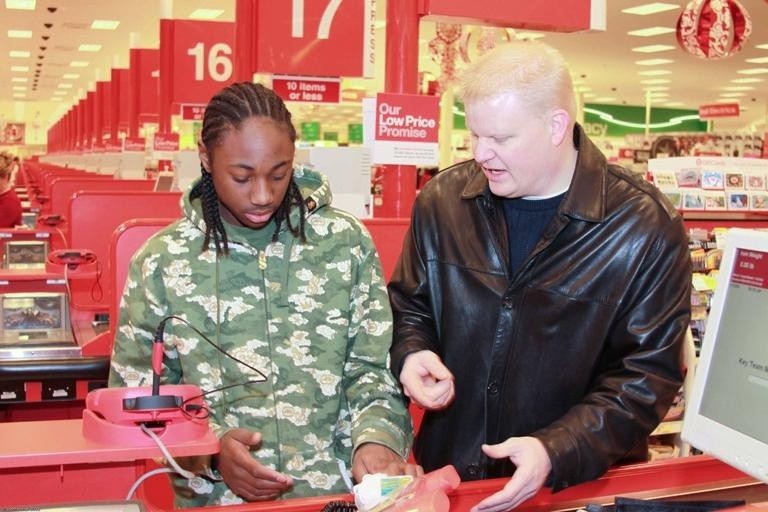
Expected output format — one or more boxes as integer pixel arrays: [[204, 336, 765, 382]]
[[109, 83, 424, 509], [0, 157, 21, 263], [386, 41, 691, 512]]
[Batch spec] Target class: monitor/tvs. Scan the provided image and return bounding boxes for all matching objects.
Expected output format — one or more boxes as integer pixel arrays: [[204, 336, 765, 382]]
[[679, 224, 768, 483]]
